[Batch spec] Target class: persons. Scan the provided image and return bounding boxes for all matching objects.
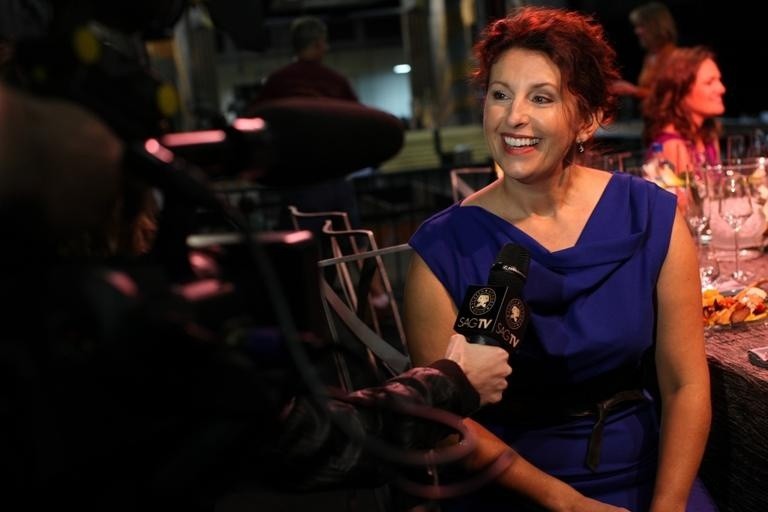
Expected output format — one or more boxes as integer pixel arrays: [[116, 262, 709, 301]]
[[636, 48, 734, 232], [0, 1, 515, 509], [396, 1, 722, 512], [606, 1, 685, 149]]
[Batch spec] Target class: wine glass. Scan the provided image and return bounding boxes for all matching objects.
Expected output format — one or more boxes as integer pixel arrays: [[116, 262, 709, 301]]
[[580, 130, 768, 293]]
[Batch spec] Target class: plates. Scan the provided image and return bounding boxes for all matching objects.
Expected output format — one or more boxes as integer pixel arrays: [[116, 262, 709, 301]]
[[701, 289, 768, 331]]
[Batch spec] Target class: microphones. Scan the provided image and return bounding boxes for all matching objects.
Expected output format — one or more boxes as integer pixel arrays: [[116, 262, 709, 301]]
[[454, 241, 531, 352]]
